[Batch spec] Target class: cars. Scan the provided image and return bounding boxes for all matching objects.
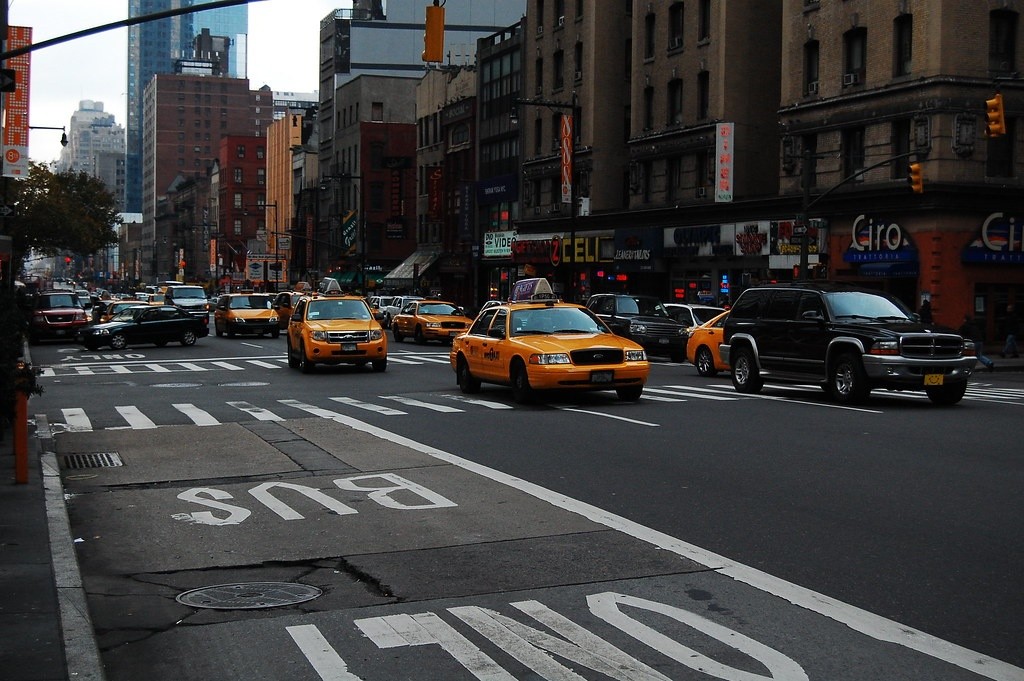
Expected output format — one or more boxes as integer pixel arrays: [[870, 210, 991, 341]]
[[686, 309, 735, 376], [78, 303, 208, 354], [586, 294, 688, 364], [272, 280, 311, 325], [653, 303, 726, 328], [450, 277, 651, 404], [36, 281, 165, 316], [213, 291, 279, 339], [368, 296, 473, 342]]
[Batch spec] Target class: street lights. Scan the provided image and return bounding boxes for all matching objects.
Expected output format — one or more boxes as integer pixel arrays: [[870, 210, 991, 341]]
[[510, 98, 577, 292], [244, 203, 280, 292], [319, 173, 365, 287]]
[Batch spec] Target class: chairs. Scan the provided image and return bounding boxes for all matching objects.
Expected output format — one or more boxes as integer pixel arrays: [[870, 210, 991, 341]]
[[513, 318, 523, 330]]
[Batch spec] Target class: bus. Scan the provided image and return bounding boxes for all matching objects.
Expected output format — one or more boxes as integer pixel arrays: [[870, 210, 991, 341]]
[[157, 281, 184, 292]]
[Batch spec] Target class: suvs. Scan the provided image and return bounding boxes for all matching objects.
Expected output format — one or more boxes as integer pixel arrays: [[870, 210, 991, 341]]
[[286, 277, 387, 374], [719, 282, 977, 408], [164, 286, 208, 320], [30, 293, 88, 341]]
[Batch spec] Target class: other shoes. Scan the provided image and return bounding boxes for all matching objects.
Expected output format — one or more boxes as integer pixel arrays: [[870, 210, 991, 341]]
[[998, 351, 1006, 359], [989, 362, 994, 372], [1010, 353, 1019, 358]]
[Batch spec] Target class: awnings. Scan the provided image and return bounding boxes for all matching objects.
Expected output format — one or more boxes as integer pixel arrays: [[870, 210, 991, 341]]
[[383, 249, 443, 279]]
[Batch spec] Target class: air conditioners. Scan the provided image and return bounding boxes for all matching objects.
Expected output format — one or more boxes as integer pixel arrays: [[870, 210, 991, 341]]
[[538, 26, 544, 33], [559, 16, 564, 26], [699, 187, 707, 196], [669, 37, 682, 49], [554, 202, 561, 212], [535, 206, 540, 214], [808, 82, 818, 93], [844, 72, 860, 85], [575, 72, 582, 81]]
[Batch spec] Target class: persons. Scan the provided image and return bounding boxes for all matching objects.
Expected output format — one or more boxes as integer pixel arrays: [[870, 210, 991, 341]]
[[958, 314, 994, 372], [1000, 305, 1020, 359]]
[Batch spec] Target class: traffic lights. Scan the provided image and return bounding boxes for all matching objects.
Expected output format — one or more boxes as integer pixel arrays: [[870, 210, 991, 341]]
[[909, 162, 923, 194], [984, 94, 1006, 138], [66, 254, 72, 267]]
